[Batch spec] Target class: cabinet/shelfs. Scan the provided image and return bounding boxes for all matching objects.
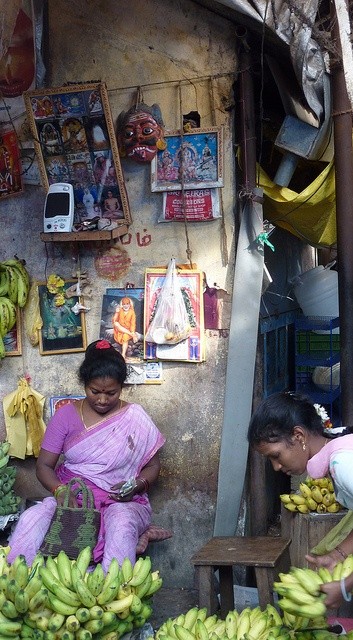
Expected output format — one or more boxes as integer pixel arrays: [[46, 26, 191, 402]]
[[293, 313, 343, 429]]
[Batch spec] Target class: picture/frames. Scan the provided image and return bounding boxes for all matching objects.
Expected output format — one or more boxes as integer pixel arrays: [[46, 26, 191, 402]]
[[35, 278, 88, 356], [23, 81, 132, 225], [150, 126, 224, 193], [2, 304, 23, 357]]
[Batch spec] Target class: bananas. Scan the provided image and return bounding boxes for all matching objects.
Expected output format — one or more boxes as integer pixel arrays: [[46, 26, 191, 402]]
[[0, 544, 162, 640], [0, 442, 22, 515], [165, 319, 191, 341], [310, 509, 353, 556], [0, 257, 30, 311], [144, 548, 353, 639], [280, 476, 340, 514], [0, 296, 18, 360]]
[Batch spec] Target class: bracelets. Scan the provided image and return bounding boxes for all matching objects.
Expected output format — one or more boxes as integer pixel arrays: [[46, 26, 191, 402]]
[[340, 577, 352, 602], [335, 547, 348, 559], [135, 476, 150, 493]]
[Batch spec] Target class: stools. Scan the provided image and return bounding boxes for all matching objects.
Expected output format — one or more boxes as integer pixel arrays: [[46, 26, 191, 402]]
[[191, 536, 293, 618]]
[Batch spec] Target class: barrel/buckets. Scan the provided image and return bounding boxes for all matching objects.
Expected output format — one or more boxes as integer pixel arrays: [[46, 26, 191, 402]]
[[289, 266, 340, 335]]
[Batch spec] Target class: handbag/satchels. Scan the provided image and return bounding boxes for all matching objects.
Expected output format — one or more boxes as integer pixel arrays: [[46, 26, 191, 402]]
[[38, 480, 101, 564]]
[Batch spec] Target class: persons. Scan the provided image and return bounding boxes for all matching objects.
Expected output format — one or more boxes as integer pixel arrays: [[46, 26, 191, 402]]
[[111, 297, 139, 360], [247, 391, 353, 609], [83, 188, 98, 218], [102, 190, 124, 219], [117, 103, 164, 164], [5, 339, 167, 578]]
